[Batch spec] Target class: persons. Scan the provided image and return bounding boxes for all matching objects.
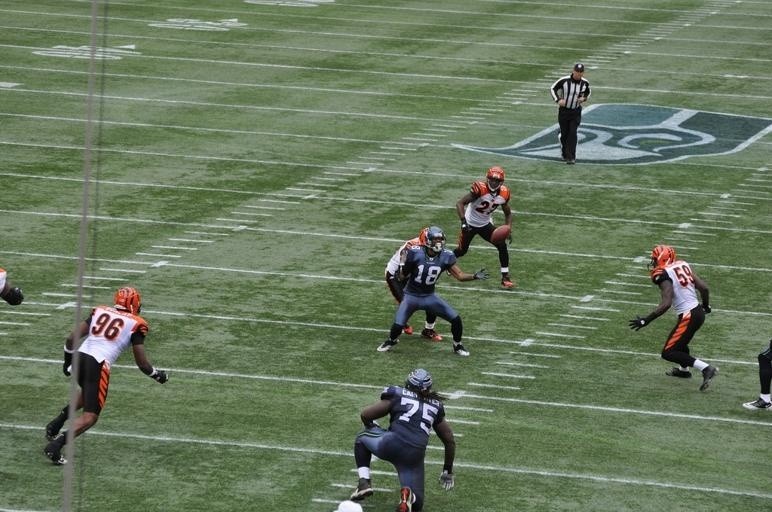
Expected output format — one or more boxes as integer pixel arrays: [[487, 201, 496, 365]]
[[445, 166, 515, 290], [42, 287, 168, 466], [627, 244, 720, 393], [741, 340, 772, 412], [383, 226, 444, 342], [0, 267, 25, 305], [550, 64, 592, 164], [375, 225, 491, 357], [348, 367, 457, 512]]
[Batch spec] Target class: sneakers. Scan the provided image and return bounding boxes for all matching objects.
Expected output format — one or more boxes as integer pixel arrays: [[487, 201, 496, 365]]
[[500, 271, 514, 290], [420, 327, 443, 342], [741, 397, 772, 411], [697, 365, 718, 393], [664, 367, 692, 378], [376, 339, 400, 352], [45, 420, 60, 441], [43, 444, 68, 466], [452, 342, 470, 357], [350, 476, 373, 502], [403, 323, 413, 336], [396, 485, 415, 512]]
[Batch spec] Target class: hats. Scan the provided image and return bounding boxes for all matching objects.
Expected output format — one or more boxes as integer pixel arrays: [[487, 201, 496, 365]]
[[573, 63, 585, 73]]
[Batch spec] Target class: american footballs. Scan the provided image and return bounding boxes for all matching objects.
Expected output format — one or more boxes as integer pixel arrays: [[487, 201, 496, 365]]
[[492, 225, 512, 241]]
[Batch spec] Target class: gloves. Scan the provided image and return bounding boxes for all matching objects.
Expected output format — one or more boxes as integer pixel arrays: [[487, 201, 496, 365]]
[[629, 316, 648, 332], [63, 345, 72, 377], [438, 470, 454, 491], [460, 217, 473, 233], [475, 268, 490, 281], [1, 282, 24, 307], [148, 365, 169, 384], [701, 304, 711, 314]]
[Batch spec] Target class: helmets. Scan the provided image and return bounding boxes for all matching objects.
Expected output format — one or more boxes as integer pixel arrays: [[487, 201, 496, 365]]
[[419, 227, 429, 245], [486, 166, 506, 192], [113, 287, 141, 316], [647, 244, 675, 272], [426, 226, 445, 253], [406, 367, 432, 391]]
